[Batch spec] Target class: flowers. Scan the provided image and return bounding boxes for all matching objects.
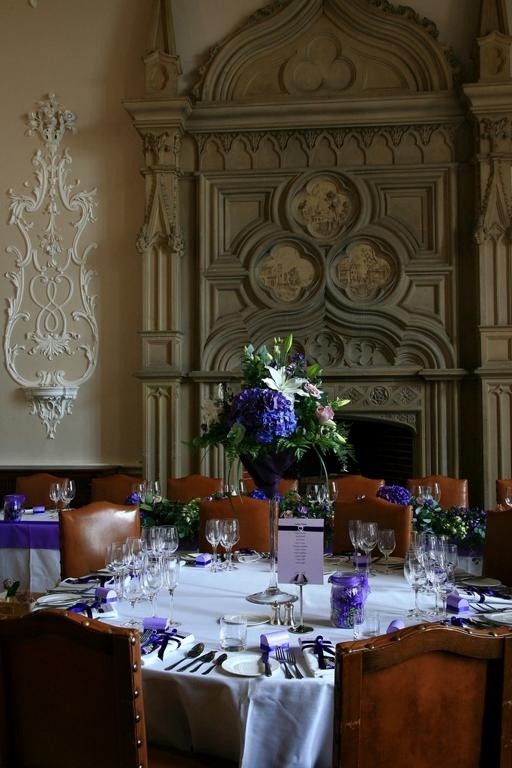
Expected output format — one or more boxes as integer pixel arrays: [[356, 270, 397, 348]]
[[182, 333, 355, 496]]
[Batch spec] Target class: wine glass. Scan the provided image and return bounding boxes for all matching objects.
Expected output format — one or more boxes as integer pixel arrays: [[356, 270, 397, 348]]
[[219, 519, 240, 571], [106, 524, 182, 629], [49, 478, 75, 519], [347, 516, 458, 619], [305, 481, 338, 507], [205, 518, 225, 573], [134, 480, 160, 503]]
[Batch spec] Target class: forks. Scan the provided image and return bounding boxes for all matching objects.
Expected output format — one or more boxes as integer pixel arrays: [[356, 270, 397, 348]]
[[275, 646, 294, 678], [140, 629, 154, 642], [467, 602, 512, 613], [47, 585, 94, 594], [284, 649, 305, 678]]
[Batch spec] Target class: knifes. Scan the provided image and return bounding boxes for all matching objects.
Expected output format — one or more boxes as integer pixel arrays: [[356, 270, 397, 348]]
[[90, 570, 110, 574], [262, 652, 272, 678], [181, 552, 196, 558], [292, 647, 314, 678]]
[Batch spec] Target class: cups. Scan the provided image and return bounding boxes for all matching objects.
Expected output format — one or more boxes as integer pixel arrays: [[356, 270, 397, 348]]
[[351, 612, 380, 640], [218, 480, 246, 496], [415, 482, 441, 503], [218, 614, 248, 652], [503, 487, 512, 509]]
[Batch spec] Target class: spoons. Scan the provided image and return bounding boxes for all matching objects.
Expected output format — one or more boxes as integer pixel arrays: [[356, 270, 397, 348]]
[[165, 643, 227, 674]]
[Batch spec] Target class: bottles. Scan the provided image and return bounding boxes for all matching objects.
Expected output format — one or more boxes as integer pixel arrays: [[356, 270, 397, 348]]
[[328, 572, 364, 629], [3, 495, 24, 520], [270, 600, 283, 626], [284, 602, 296, 627]]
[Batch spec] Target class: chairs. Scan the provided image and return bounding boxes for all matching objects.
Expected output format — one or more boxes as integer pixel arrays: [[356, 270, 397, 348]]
[[6, 468, 512, 586], [1, 610, 148, 768], [334, 623, 512, 768]]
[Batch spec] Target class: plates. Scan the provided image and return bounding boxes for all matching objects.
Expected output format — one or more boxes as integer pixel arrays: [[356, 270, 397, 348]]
[[461, 578, 501, 587], [97, 569, 112, 577], [479, 614, 511, 626], [223, 613, 270, 626], [38, 592, 82, 606], [179, 552, 204, 561], [220, 654, 279, 676]]
